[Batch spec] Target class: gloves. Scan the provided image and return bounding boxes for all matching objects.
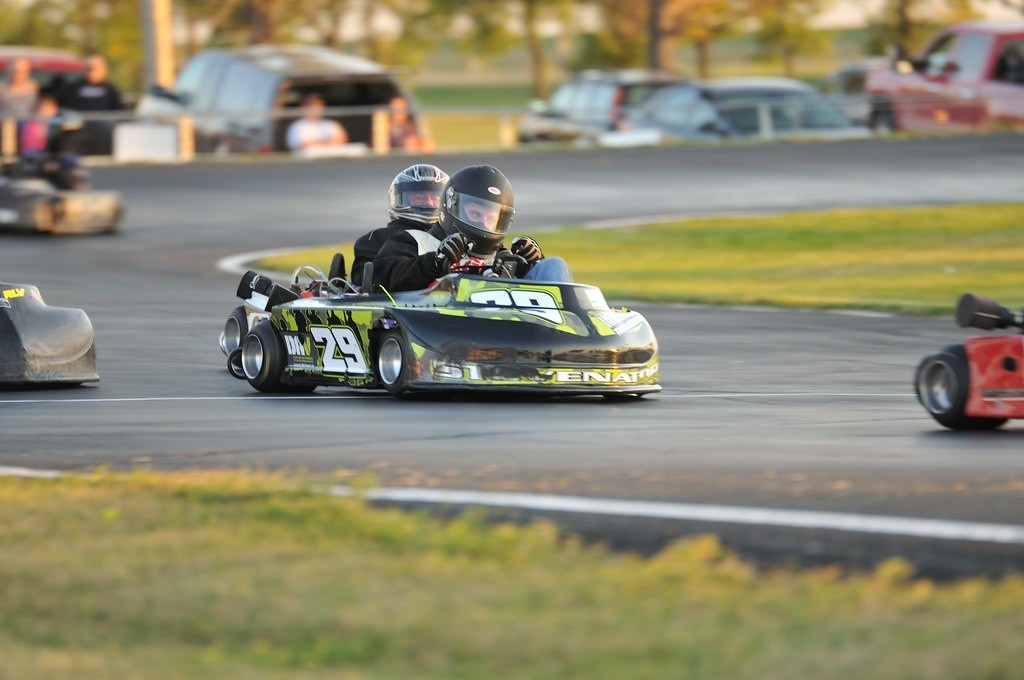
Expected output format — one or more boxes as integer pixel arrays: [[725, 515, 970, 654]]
[[511, 233, 544, 264], [438, 232, 476, 263]]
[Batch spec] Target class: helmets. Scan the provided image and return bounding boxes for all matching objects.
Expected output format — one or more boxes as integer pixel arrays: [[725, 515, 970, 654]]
[[439, 164, 514, 258], [389, 164, 451, 225]]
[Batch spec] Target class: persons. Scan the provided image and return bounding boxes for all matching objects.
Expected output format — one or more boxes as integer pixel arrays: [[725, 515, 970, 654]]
[[283, 94, 347, 152], [372, 164, 545, 294], [351, 164, 450, 286], [0, 55, 127, 155], [385, 97, 435, 155]]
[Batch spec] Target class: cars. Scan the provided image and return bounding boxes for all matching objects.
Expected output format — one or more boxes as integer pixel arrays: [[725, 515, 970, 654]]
[[2, 43, 91, 157], [512, 20, 1024, 147]]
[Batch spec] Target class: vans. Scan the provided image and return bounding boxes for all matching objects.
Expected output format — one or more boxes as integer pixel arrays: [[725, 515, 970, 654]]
[[167, 40, 428, 156]]
